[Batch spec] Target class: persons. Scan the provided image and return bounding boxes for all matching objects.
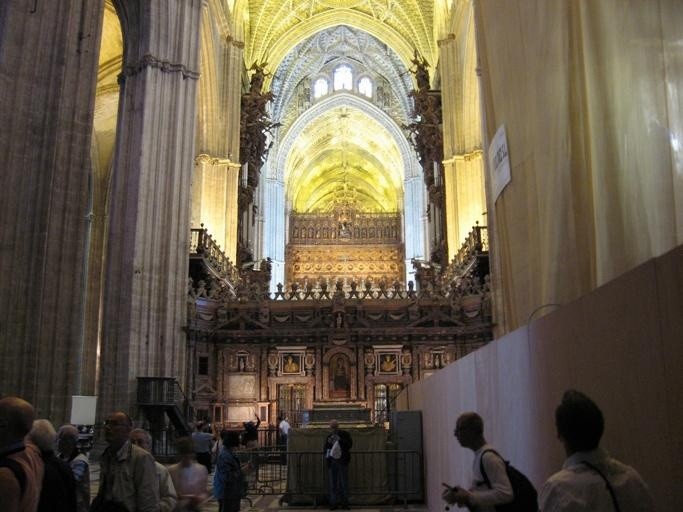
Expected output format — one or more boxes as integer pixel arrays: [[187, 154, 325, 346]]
[[436, 413, 515, 511], [323, 418, 353, 510], [334, 356, 346, 389], [193, 422, 218, 474], [89, 411, 160, 512], [214, 430, 253, 512], [129, 427, 176, 511], [242, 413, 260, 468], [277, 415, 290, 465], [54, 423, 89, 510], [28, 418, 76, 511], [538, 388, 659, 511], [0, 396, 44, 511], [168, 436, 209, 512]]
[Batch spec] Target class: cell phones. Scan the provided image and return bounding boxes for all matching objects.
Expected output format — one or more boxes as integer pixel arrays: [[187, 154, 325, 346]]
[[442, 482, 453, 490]]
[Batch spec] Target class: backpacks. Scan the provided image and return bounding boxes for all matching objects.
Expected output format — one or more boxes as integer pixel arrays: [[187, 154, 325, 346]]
[[478, 448, 539, 512]]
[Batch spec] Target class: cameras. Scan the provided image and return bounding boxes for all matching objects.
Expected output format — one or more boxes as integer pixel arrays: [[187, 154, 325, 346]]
[[329, 435, 337, 441]]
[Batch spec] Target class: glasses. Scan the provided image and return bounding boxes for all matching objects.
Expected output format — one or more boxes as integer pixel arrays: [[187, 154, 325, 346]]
[[103, 420, 129, 427]]
